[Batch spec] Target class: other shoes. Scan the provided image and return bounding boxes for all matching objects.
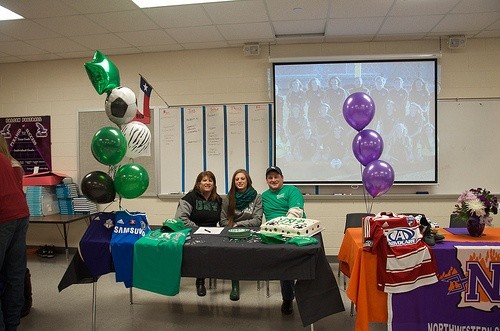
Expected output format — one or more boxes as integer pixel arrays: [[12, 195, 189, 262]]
[[196, 279, 206, 296], [281, 299, 293, 315]]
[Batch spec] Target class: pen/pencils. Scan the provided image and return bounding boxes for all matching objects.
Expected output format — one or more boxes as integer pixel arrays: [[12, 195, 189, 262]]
[[204, 229, 210, 233]]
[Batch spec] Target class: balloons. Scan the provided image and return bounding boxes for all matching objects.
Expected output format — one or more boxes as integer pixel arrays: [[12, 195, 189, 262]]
[[362, 160, 395, 197], [114, 164, 150, 199], [122, 121, 151, 158], [106, 86, 138, 124], [343, 92, 375, 131], [352, 129, 384, 167], [81, 170, 117, 204], [91, 127, 128, 165], [83, 50, 120, 95]]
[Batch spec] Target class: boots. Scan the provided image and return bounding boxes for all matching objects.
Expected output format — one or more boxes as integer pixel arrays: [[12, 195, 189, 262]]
[[230, 280, 240, 301]]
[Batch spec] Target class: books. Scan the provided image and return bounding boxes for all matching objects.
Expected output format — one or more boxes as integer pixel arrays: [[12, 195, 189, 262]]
[[26, 183, 96, 216]]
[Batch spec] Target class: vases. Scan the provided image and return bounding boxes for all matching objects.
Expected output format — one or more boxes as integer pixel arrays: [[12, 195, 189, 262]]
[[466, 216, 485, 237]]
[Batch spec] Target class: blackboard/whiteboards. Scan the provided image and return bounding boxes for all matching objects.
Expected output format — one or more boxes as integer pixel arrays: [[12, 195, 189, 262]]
[[295, 96, 500, 201], [159, 102, 274, 199]]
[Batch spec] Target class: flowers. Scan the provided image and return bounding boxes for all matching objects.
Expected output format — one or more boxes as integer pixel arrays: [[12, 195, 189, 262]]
[[454, 188, 499, 226]]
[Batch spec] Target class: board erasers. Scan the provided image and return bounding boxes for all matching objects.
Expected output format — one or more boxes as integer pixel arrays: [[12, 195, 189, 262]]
[[416, 191, 428, 194]]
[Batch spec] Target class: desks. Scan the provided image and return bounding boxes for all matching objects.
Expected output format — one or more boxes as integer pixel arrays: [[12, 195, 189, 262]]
[[29, 213, 93, 264], [57, 226, 345, 331], [336, 228, 500, 331]]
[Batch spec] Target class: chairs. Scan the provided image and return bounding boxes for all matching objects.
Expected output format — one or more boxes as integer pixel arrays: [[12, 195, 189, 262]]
[[337, 213, 375, 290], [449, 213, 467, 229]]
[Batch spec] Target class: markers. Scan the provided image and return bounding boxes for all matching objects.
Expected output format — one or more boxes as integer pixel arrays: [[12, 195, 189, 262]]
[[333, 193, 343, 195], [168, 192, 184, 195], [302, 193, 310, 196]]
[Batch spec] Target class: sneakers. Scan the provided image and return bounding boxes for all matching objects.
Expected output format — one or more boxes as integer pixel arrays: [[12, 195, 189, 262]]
[[37, 244, 57, 258]]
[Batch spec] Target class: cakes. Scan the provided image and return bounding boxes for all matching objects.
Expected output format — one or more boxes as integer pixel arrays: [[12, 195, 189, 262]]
[[259, 216, 321, 237]]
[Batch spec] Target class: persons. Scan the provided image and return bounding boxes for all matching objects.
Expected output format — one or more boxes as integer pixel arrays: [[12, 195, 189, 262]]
[[262, 166, 307, 312], [0, 135, 30, 331], [275, 76, 435, 169], [220, 169, 263, 300], [175, 171, 222, 297]]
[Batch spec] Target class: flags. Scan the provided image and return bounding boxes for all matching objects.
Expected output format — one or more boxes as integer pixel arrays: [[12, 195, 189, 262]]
[[138, 77, 153, 116]]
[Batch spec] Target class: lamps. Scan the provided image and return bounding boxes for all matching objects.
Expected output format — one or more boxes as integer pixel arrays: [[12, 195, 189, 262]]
[[449, 35, 466, 48], [242, 42, 260, 56]]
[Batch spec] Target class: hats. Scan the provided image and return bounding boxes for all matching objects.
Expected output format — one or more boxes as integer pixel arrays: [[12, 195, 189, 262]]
[[265, 166, 283, 180]]
